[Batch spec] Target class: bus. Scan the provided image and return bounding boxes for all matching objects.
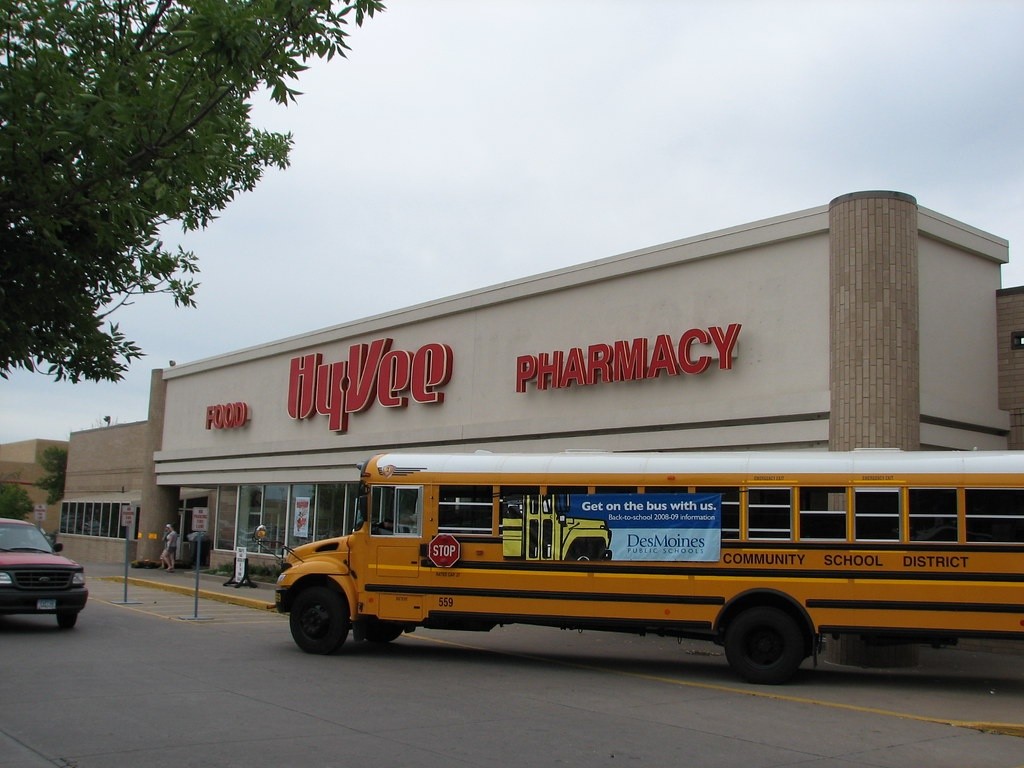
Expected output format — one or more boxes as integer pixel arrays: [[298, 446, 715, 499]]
[[251, 449, 1024, 687]]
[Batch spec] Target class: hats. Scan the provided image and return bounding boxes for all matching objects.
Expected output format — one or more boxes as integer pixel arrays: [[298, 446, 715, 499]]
[[165, 523, 171, 528]]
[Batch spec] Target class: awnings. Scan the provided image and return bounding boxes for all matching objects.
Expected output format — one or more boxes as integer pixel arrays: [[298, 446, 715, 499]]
[[62, 488, 208, 503]]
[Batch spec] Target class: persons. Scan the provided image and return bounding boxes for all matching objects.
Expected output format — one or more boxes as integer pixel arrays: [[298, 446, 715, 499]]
[[159, 523, 177, 572]]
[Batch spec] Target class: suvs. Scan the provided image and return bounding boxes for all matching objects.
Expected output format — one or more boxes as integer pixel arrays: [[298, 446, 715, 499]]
[[0, 519, 90, 629]]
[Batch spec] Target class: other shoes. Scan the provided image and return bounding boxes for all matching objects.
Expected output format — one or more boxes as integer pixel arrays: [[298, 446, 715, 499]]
[[166, 566, 174, 573], [158, 566, 164, 570]]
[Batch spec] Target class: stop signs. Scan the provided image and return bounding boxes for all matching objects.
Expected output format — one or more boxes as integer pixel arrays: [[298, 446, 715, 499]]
[[428, 533, 463, 569]]
